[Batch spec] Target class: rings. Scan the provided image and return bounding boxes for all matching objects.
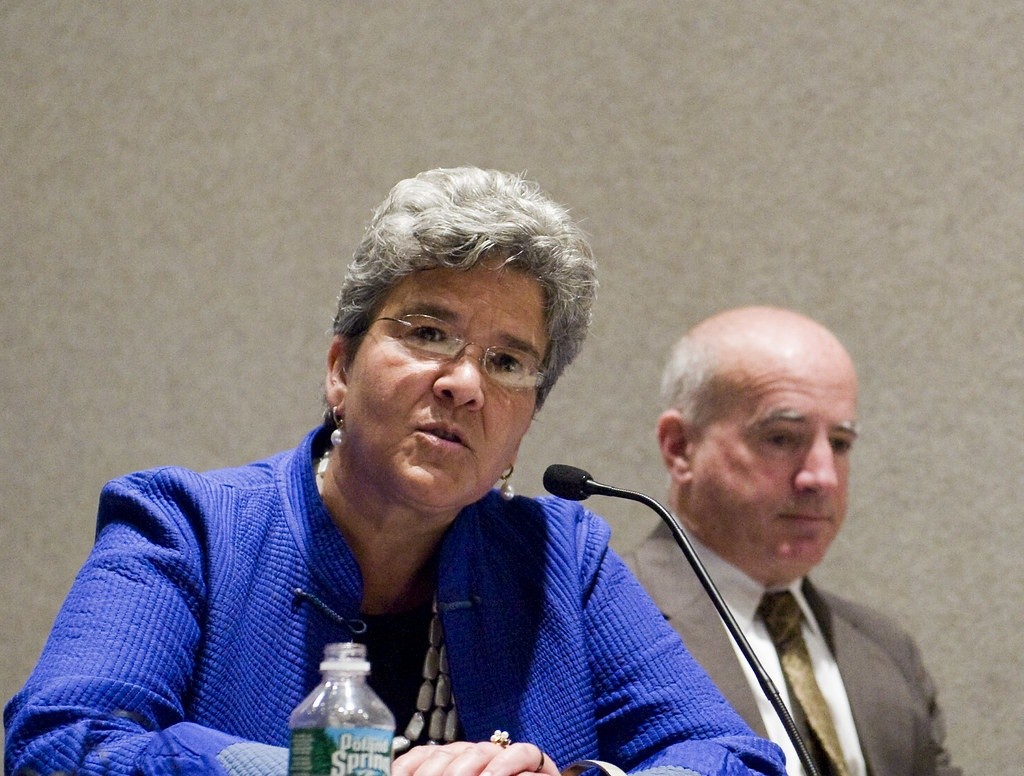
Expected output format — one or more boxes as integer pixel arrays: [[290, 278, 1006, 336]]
[[535, 750, 545, 774], [490, 729, 511, 748]]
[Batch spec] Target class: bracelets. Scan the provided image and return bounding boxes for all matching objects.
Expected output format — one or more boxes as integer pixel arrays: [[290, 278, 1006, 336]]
[[560, 759, 626, 776]]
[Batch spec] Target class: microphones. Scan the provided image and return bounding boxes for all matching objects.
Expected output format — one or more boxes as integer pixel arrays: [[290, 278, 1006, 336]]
[[542, 463, 821, 776]]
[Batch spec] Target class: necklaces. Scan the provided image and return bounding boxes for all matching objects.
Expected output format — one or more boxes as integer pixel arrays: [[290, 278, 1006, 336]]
[[310, 448, 462, 764]]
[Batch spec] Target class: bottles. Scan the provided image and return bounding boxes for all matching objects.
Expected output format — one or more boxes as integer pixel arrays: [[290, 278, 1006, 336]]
[[286, 643, 395, 776]]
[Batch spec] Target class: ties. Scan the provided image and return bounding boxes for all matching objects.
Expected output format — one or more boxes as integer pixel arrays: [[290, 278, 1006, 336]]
[[756, 589, 849, 776]]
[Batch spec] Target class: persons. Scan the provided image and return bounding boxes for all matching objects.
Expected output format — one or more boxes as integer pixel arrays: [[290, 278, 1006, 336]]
[[2, 163, 790, 775], [616, 304, 970, 776]]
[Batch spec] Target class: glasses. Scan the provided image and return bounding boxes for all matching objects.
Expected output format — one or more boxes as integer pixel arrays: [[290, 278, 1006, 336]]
[[360, 314, 549, 390]]
